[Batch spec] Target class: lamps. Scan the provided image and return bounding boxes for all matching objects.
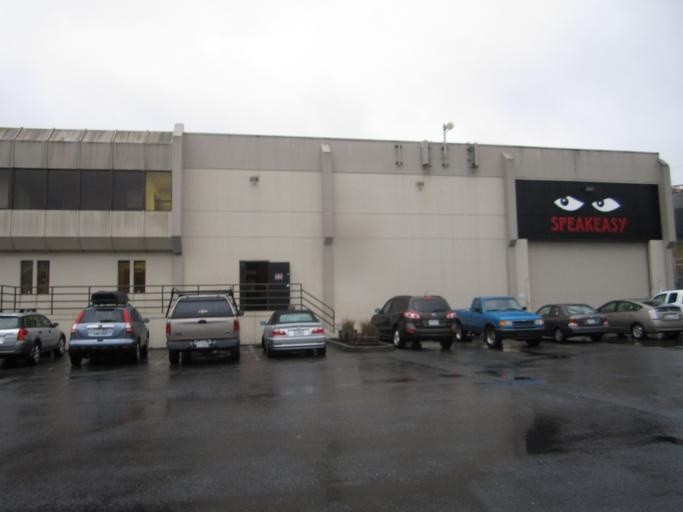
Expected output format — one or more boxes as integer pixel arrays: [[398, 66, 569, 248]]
[[443, 122, 453, 167]]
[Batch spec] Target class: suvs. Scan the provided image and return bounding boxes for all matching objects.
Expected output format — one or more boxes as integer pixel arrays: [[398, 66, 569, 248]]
[[165, 293, 241, 366], [67, 289, 149, 364], [371, 295, 457, 348]]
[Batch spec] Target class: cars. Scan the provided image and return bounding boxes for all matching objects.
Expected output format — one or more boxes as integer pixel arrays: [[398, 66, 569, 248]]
[[259, 309, 327, 356], [534, 303, 609, 344], [596, 298, 683, 339], [0, 311, 66, 364]]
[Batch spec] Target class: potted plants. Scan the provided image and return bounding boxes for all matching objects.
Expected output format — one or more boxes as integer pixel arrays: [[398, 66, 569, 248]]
[[338, 320, 380, 345]]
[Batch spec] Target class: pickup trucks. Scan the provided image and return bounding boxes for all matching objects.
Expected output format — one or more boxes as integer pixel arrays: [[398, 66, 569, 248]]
[[650, 289, 683, 312], [452, 296, 546, 348]]
